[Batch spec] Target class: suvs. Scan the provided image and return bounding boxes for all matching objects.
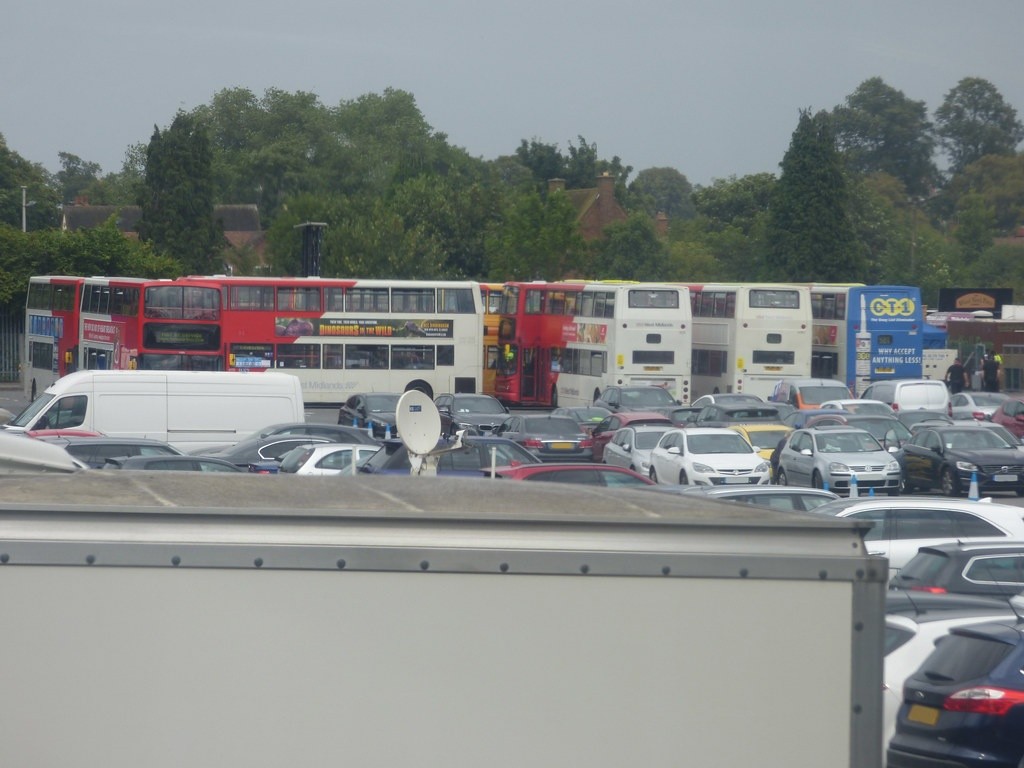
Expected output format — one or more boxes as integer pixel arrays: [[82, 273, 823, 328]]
[[13, 430, 241, 473], [595, 384, 681, 413], [804, 498, 1024, 768], [432, 391, 510, 437], [911, 413, 1024, 465]]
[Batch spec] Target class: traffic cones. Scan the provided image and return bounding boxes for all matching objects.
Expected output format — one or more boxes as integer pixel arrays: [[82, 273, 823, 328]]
[[967, 470, 980, 501]]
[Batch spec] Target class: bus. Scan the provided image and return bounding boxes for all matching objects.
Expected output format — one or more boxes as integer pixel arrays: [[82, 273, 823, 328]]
[[187, 275, 483, 409], [21, 275, 225, 403], [479, 283, 813, 412], [807, 282, 926, 400]]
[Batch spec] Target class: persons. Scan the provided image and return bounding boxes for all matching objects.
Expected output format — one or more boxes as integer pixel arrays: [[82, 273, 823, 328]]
[[944, 358, 970, 395], [980, 348, 1001, 392]]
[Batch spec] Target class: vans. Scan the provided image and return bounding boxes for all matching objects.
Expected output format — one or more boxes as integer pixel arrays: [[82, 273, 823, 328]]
[[0, 369, 304, 448], [861, 378, 953, 419], [773, 377, 854, 412]]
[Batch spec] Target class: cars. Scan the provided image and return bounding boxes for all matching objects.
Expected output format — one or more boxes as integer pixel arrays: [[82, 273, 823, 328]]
[[187, 423, 540, 478], [689, 391, 796, 486], [337, 393, 402, 438], [479, 407, 771, 490], [950, 391, 1024, 440], [699, 412, 904, 513], [892, 428, 1024, 498]]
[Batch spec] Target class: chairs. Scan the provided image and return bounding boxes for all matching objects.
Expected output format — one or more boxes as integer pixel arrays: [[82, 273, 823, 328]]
[[816, 436, 826, 451]]
